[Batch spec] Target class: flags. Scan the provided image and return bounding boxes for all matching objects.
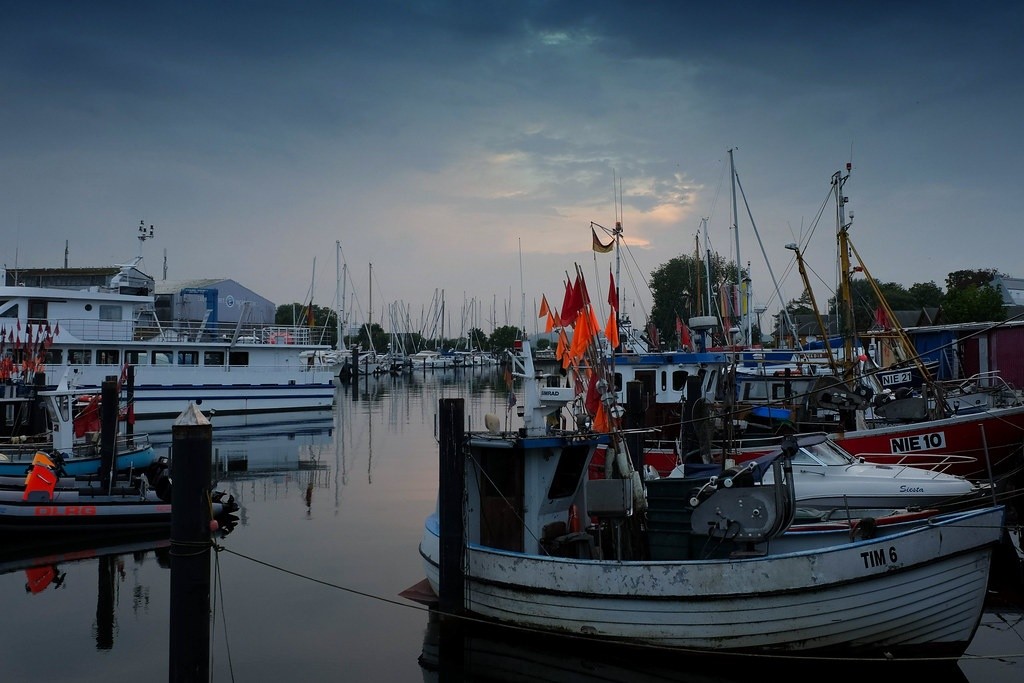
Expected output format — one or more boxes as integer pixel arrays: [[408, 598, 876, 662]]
[[74, 371, 135, 438], [0, 320, 60, 383], [650, 317, 691, 348], [539, 265, 619, 433], [592, 229, 615, 253]]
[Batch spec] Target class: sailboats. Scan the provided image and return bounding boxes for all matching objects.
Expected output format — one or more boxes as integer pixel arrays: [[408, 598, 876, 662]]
[[299, 240, 499, 377], [566, 148, 1024, 483]]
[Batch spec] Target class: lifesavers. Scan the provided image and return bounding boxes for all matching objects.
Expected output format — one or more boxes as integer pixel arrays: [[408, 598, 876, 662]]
[[78, 394, 101, 403]]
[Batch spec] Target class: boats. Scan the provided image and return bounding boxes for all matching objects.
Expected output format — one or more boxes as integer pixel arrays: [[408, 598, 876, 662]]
[[0, 286, 334, 445], [0, 380, 239, 529], [419, 339, 1005, 660]]
